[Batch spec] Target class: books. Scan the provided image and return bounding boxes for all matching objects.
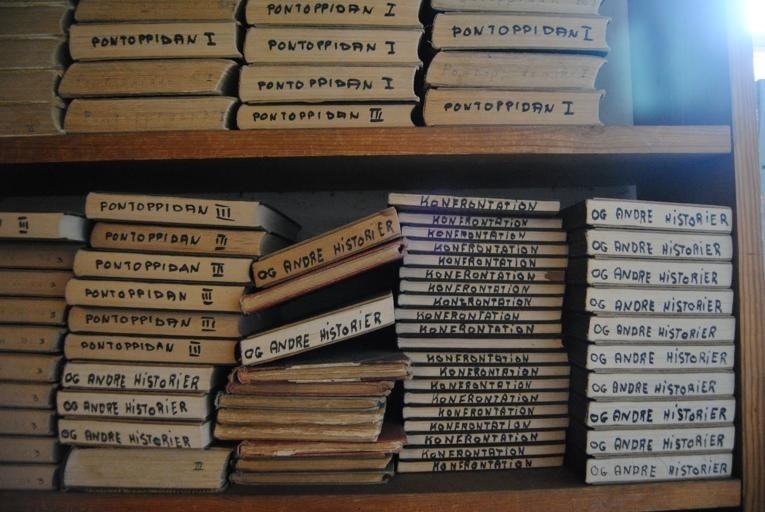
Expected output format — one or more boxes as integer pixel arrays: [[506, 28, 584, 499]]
[[1, 191, 409, 494], [562, 196, 736, 485], [1, 0, 610, 137], [388, 192, 570, 474]]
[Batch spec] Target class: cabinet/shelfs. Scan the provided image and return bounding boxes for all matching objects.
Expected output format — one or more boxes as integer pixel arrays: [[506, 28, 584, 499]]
[[0, 1, 765, 512]]
